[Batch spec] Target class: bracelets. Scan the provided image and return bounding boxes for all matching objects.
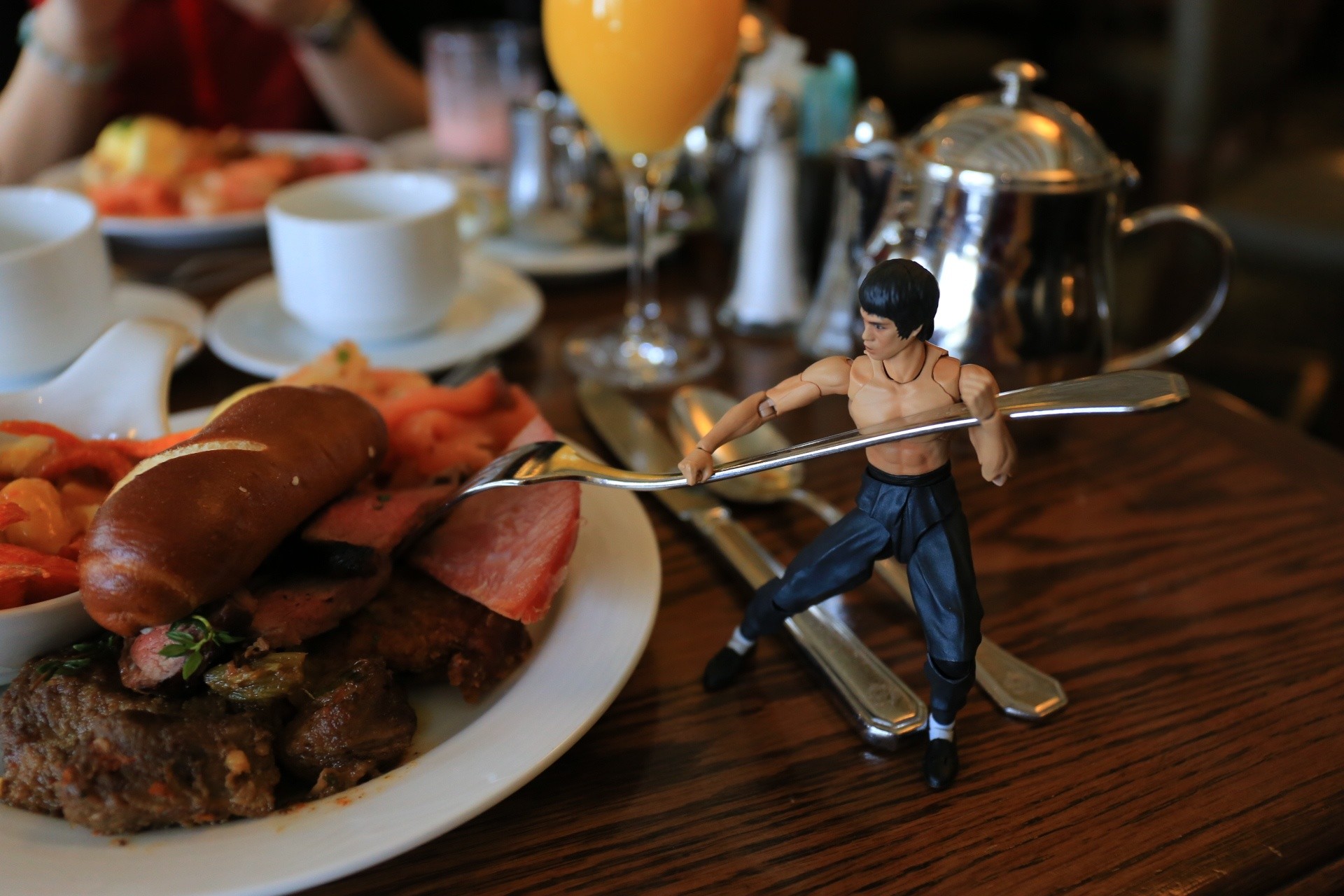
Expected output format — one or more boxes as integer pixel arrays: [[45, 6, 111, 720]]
[[291, 4, 363, 57], [14, 7, 125, 90]]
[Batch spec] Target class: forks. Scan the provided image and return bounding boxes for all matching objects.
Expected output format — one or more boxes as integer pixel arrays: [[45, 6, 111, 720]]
[[442, 368, 1190, 505]]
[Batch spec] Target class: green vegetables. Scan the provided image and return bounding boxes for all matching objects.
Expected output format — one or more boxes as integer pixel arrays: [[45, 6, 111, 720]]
[[25, 347, 473, 683], [73, 382, 390, 631]]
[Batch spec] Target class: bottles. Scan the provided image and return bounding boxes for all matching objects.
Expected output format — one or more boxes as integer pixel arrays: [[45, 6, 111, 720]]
[[713, 92, 815, 338], [791, 100, 901, 359]]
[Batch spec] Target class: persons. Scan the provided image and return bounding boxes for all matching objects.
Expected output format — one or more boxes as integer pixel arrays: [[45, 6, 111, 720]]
[[676, 264, 1014, 789], [0, 0, 431, 185]]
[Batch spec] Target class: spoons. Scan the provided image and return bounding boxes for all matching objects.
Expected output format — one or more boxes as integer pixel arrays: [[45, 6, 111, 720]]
[[669, 384, 1069, 720]]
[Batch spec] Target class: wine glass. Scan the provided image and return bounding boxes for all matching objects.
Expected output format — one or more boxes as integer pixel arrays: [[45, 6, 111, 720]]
[[541, 0, 750, 390], [429, 26, 536, 247]]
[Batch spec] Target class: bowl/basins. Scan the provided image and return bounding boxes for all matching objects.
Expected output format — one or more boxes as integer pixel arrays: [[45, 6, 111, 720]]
[[1, 184, 115, 394], [265, 169, 465, 345], [1, 589, 88, 689]]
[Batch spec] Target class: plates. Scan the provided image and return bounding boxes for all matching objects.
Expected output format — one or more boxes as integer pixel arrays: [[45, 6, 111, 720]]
[[109, 275, 208, 368], [470, 210, 678, 275], [0, 422, 663, 896], [27, 129, 380, 242], [203, 250, 546, 379]]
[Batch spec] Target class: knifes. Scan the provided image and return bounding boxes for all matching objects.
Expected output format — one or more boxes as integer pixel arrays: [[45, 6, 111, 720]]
[[573, 377, 930, 743]]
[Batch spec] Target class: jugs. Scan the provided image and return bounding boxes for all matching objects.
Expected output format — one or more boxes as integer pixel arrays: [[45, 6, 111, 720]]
[[845, 57, 1236, 452]]
